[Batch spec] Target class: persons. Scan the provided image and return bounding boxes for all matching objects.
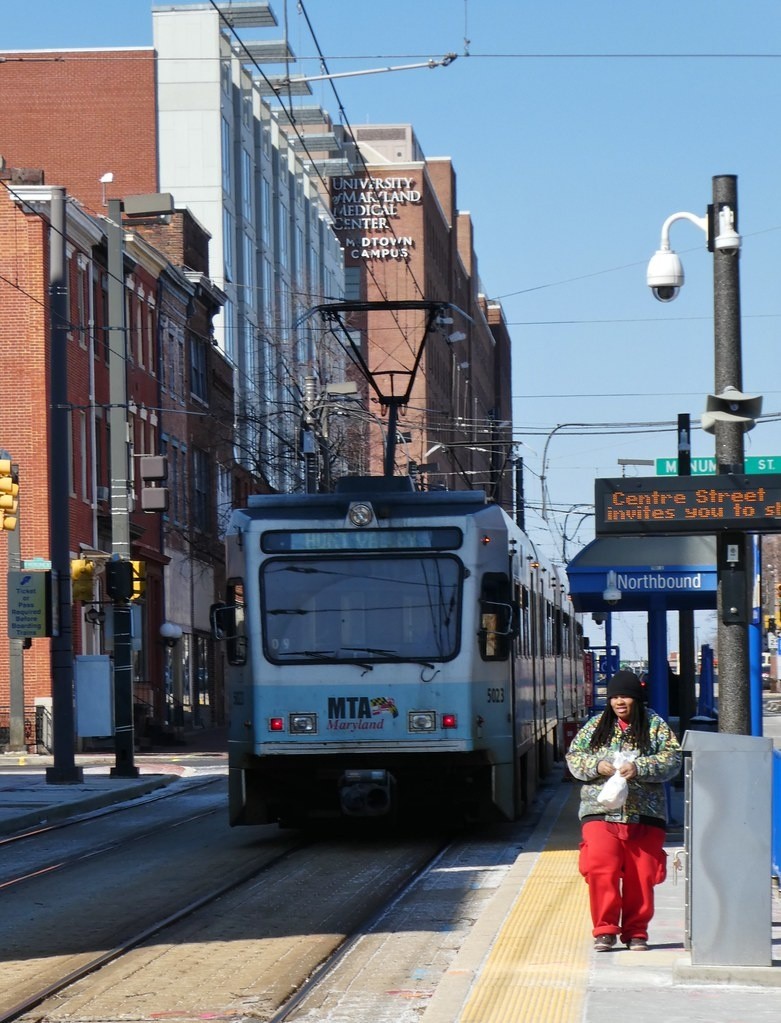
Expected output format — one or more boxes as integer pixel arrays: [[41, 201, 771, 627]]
[[565, 672, 685, 952]]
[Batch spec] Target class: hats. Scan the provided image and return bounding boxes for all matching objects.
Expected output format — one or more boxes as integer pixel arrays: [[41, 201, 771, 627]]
[[607, 671, 642, 699]]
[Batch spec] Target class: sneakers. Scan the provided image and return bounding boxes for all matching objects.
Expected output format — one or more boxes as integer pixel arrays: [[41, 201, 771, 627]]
[[626, 938, 649, 951], [593, 934, 617, 951]]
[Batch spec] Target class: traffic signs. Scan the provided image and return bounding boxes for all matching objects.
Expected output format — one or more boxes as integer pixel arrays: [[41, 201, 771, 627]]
[[595, 473, 781, 537]]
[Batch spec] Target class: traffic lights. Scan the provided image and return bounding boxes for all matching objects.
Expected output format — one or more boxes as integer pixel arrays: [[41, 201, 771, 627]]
[[769, 618, 775, 632]]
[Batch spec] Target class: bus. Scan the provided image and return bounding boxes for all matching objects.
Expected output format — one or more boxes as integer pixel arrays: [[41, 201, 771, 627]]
[[214, 476, 590, 828]]
